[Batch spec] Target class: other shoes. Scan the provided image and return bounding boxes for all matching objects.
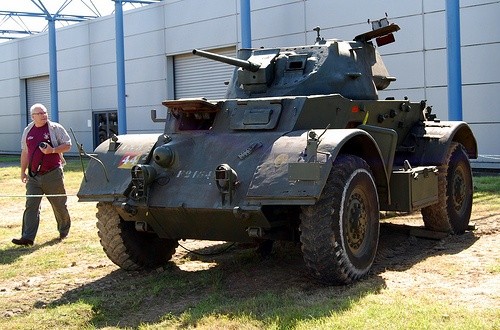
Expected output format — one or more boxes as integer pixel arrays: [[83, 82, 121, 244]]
[[12, 238, 34, 246], [60, 218, 70, 239]]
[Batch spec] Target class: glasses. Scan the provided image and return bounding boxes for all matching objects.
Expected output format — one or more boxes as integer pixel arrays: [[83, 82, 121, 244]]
[[32, 112, 48, 116]]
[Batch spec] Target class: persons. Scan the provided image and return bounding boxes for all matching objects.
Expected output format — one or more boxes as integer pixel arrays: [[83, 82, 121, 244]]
[[11, 103, 72, 246]]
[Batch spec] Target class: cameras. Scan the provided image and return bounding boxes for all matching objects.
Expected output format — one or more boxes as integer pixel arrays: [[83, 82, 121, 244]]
[[39, 140, 51, 149]]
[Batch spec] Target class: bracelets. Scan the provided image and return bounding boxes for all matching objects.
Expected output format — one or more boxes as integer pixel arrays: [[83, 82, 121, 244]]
[[52, 148, 55, 153]]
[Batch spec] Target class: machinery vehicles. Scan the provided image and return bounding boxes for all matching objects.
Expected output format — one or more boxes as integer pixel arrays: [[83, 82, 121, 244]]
[[69, 13, 480, 287]]
